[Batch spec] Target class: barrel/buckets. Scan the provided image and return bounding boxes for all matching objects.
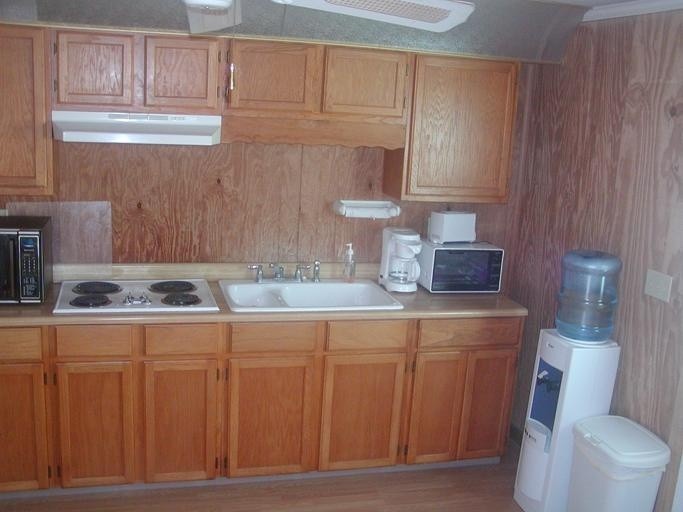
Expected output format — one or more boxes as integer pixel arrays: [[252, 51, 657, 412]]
[[554, 249, 623, 342]]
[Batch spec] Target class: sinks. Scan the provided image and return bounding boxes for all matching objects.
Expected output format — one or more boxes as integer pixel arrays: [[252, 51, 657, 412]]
[[281, 278, 404, 313], [218, 278, 285, 312]]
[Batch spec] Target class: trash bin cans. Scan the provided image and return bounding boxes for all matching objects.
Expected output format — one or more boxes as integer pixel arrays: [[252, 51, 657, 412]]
[[565, 414, 671, 512]]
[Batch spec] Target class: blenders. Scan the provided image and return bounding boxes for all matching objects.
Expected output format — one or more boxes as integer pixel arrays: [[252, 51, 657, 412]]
[[378, 227, 423, 293]]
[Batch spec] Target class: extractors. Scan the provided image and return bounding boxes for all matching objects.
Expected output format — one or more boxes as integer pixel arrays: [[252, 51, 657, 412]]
[[50, 111, 222, 146]]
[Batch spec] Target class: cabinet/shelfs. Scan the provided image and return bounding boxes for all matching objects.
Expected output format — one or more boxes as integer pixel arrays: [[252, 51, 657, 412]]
[[225, 39, 411, 151], [384, 54, 522, 204], [0, 28, 53, 198], [57, 29, 224, 113], [0, 308, 527, 496]]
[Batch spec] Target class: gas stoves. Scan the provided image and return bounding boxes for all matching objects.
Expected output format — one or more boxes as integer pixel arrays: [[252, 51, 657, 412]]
[[53, 278, 220, 313]]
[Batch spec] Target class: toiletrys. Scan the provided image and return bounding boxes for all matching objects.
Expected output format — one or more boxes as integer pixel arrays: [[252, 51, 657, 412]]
[[342, 243, 357, 284]]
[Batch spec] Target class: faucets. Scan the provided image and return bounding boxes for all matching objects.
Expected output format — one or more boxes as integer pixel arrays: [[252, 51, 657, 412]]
[[248, 262, 264, 283], [312, 260, 321, 283], [294, 263, 312, 283], [269, 264, 283, 281]]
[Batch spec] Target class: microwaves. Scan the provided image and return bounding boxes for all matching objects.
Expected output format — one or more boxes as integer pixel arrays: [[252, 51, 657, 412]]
[[420, 241, 505, 295]]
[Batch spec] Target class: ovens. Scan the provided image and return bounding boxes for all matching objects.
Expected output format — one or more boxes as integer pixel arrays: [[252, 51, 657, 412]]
[[0, 216, 53, 303]]
